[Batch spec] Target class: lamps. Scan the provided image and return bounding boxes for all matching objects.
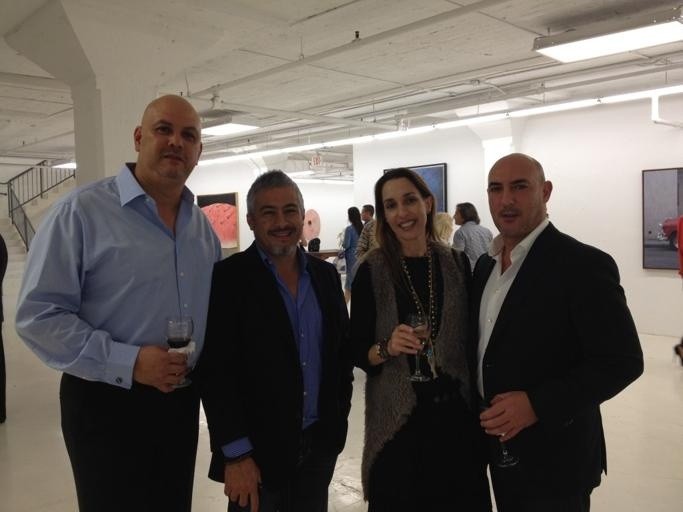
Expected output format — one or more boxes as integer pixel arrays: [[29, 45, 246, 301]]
[[201, 112, 261, 136], [530, 5, 682, 65]]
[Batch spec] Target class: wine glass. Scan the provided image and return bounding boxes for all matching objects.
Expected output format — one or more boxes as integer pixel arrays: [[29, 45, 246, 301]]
[[405, 313, 434, 384], [163, 317, 192, 390], [475, 393, 519, 469]]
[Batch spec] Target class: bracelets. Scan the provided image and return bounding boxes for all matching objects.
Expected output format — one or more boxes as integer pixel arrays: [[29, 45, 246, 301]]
[[377, 336, 398, 360]]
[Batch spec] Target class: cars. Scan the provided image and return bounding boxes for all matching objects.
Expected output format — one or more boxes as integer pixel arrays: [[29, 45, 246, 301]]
[[657, 216, 679, 249]]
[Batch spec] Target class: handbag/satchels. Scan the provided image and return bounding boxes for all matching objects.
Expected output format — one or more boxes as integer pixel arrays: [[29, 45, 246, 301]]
[[333, 245, 347, 274]]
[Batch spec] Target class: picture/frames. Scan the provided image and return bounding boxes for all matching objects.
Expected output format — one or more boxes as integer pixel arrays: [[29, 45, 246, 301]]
[[384, 162, 448, 214], [641, 167, 682, 270], [196, 191, 240, 250]]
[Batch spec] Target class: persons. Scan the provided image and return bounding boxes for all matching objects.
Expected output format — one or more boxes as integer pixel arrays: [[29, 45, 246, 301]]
[[350, 168, 493, 512], [673, 215, 683, 365], [452, 202, 493, 276], [15, 95, 223, 512], [340, 207, 364, 304], [468, 152, 644, 512], [354, 220, 380, 260], [0, 233, 9, 424], [361, 204, 374, 225], [195, 169, 354, 512], [433, 212, 455, 245]]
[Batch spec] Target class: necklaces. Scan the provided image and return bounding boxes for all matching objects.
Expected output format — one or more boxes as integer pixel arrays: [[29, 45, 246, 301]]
[[401, 239, 438, 379]]
